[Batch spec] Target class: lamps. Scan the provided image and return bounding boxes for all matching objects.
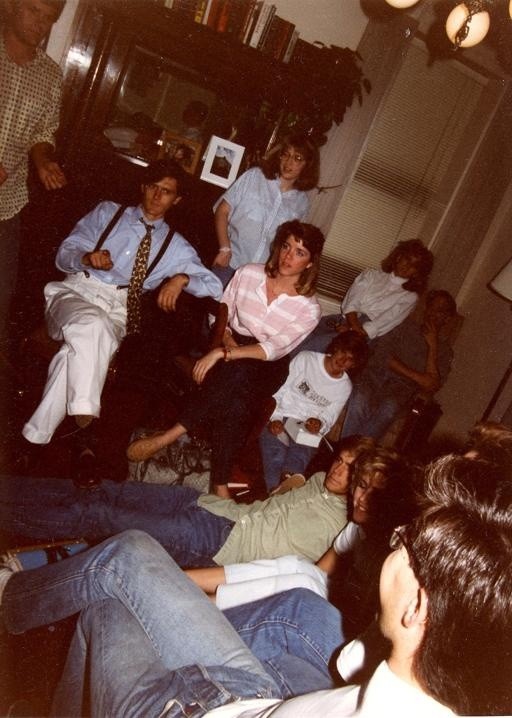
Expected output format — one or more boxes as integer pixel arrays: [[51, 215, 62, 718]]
[[446, 0, 496, 57]]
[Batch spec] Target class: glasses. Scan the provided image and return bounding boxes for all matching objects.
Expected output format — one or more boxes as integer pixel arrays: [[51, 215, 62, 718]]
[[279, 149, 303, 165], [389, 524, 409, 553]]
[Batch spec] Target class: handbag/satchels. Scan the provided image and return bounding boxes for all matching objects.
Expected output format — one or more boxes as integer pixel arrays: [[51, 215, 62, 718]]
[[128, 426, 213, 497]]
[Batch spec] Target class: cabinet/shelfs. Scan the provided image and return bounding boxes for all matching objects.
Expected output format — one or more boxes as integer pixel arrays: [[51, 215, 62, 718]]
[[48, 0, 294, 167]]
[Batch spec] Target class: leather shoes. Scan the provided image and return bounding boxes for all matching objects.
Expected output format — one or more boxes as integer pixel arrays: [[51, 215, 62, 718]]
[[72, 452, 102, 489], [127, 437, 170, 462], [13, 448, 36, 464]]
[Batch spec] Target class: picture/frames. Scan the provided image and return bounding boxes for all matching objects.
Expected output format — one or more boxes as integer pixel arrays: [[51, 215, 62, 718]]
[[158, 131, 201, 174], [200, 134, 245, 190]]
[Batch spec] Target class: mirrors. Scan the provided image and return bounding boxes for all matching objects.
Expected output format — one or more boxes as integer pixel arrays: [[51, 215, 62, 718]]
[[97, 51, 220, 175]]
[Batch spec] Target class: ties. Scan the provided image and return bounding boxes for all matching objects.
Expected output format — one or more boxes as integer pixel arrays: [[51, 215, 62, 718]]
[[126, 217, 155, 336]]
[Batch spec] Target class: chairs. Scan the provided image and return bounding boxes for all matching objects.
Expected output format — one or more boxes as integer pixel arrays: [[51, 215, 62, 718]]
[[326, 397, 442, 448], [22, 290, 227, 386]]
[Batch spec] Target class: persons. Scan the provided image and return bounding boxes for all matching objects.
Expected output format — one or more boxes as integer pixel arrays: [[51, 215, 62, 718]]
[[1, 161, 224, 474], [183, 451, 408, 611], [125, 219, 324, 503], [1, 434, 374, 567], [1, 0, 67, 261], [212, 133, 320, 291], [339, 290, 454, 450], [0, 454, 511, 716], [258, 331, 369, 495], [217, 420, 512, 699], [290, 238, 433, 358]]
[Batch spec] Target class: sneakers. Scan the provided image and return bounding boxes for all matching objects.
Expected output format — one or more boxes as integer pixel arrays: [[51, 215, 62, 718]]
[[1, 554, 23, 604], [281, 473, 306, 491], [269, 485, 283, 496]]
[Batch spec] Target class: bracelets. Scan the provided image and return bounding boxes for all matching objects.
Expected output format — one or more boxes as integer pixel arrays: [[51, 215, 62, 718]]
[[225, 345, 230, 362], [219, 246, 231, 252]]
